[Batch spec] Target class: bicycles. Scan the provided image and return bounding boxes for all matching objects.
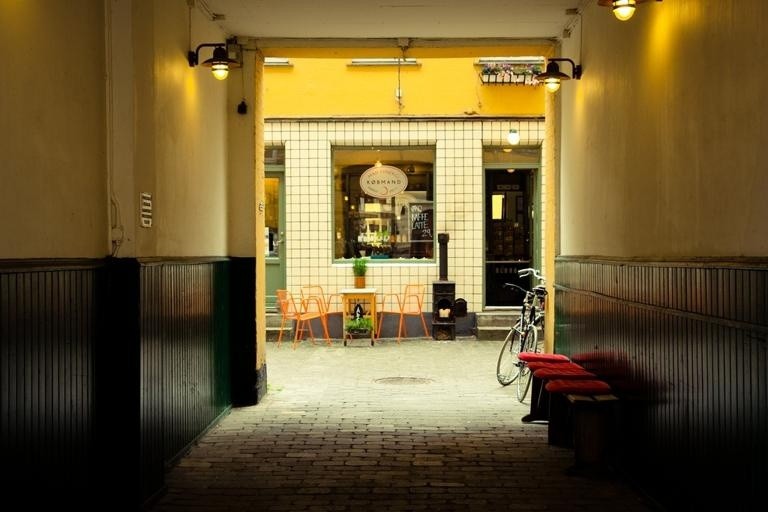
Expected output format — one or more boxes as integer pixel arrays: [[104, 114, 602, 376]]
[[497, 283, 545, 402]]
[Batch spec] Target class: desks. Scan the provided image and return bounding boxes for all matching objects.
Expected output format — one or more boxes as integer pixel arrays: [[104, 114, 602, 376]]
[[336, 288, 379, 346]]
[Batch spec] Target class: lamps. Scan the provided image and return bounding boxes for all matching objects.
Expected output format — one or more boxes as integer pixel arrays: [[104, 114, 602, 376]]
[[534, 56, 582, 94], [187, 42, 243, 81], [598, 0, 662, 21]]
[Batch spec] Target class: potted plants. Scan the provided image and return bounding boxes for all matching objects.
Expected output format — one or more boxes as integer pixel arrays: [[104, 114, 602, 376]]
[[351, 257, 368, 287], [346, 318, 371, 336]]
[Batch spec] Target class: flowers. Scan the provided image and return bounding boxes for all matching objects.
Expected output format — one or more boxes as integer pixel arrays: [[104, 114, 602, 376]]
[[482, 64, 542, 78]]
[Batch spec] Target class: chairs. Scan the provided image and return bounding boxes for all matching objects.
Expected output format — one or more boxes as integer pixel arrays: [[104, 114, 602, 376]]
[[275, 284, 340, 352], [376, 284, 432, 347]]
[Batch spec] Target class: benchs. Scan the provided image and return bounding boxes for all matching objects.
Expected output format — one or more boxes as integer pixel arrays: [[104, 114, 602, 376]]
[[518, 351, 619, 470]]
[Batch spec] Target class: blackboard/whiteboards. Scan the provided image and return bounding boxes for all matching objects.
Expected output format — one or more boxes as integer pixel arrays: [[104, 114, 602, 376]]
[[408, 201, 434, 243]]
